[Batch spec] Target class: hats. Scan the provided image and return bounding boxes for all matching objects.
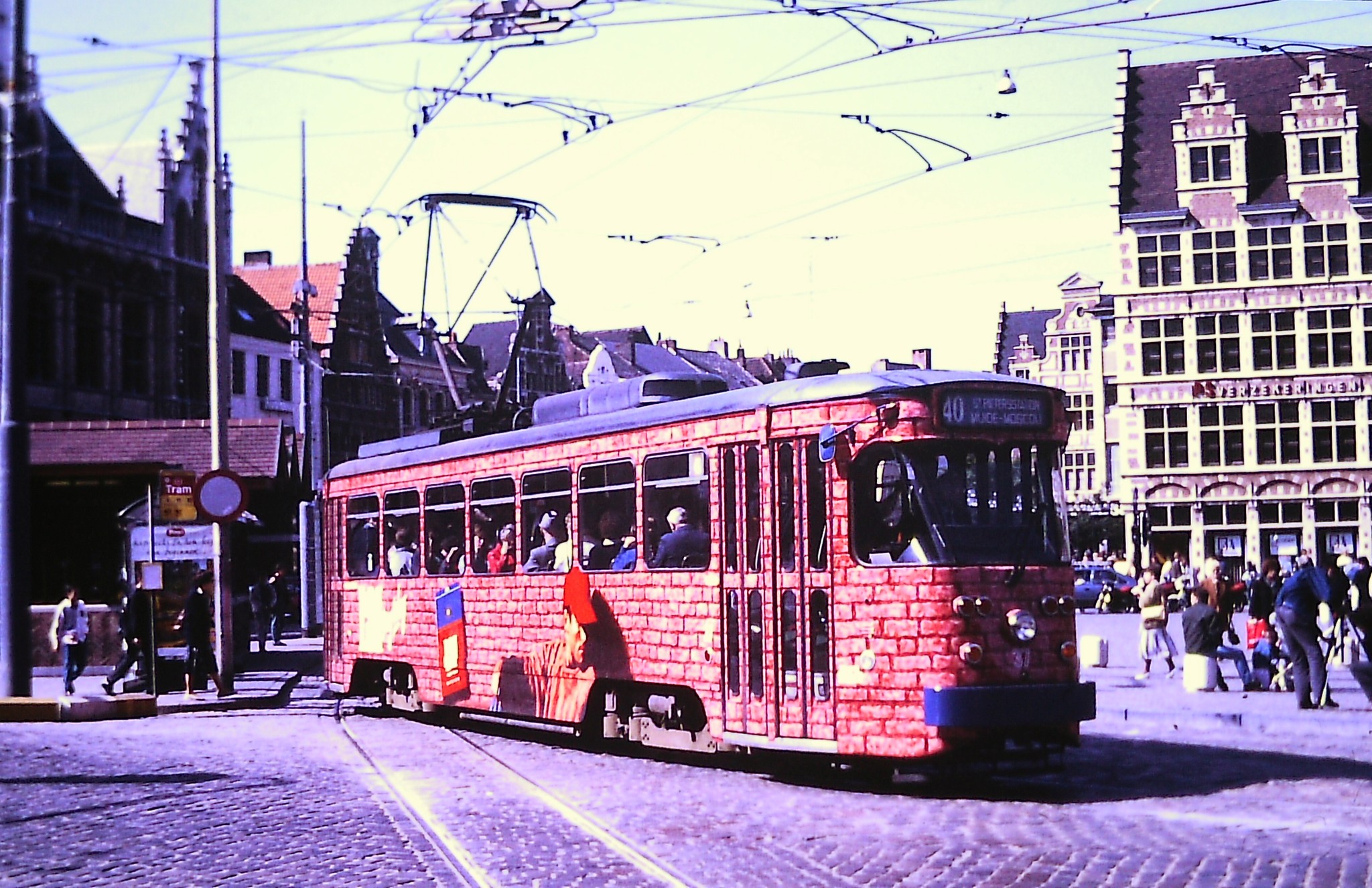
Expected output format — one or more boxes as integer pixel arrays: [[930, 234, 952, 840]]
[[538, 510, 562, 530]]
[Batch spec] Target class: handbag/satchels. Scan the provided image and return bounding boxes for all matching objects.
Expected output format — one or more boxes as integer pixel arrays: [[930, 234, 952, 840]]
[[1141, 605, 1166, 621], [1227, 620, 1240, 645], [1247, 618, 1274, 649]]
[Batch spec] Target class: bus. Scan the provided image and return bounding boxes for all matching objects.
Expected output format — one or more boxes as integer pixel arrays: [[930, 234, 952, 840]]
[[313, 193, 1096, 797]]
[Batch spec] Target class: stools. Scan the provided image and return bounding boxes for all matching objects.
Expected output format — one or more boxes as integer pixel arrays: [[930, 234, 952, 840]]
[[1183, 654, 1217, 691], [1080, 636, 1108, 667]]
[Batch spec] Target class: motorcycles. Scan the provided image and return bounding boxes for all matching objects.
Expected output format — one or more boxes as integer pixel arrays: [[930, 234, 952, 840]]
[[1230, 580, 1248, 611]]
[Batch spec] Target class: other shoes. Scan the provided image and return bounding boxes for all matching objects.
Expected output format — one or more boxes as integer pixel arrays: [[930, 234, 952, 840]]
[[1169, 667, 1176, 678], [1286, 680, 1295, 692], [274, 642, 288, 646], [101, 683, 115, 695], [65, 685, 75, 696], [1317, 697, 1339, 708], [1299, 699, 1319, 709], [1138, 671, 1149, 679], [1244, 682, 1260, 691], [1218, 683, 1229, 691]]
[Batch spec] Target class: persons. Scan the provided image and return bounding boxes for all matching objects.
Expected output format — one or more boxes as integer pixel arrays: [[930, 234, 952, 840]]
[[101, 577, 161, 696], [648, 507, 711, 569], [1071, 549, 1189, 585], [47, 584, 91, 696], [1135, 557, 1261, 692], [1248, 557, 1372, 710], [1244, 562, 1255, 599], [489, 567, 599, 722], [1252, 563, 1258, 577], [250, 569, 277, 655], [612, 520, 657, 570], [522, 510, 567, 572], [587, 510, 628, 570], [386, 508, 516, 576], [268, 561, 287, 646], [1301, 550, 1309, 564], [553, 513, 597, 571], [181, 571, 238, 701]]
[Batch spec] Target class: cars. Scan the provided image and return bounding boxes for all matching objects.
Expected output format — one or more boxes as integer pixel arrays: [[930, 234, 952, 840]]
[[1072, 566, 1137, 613]]
